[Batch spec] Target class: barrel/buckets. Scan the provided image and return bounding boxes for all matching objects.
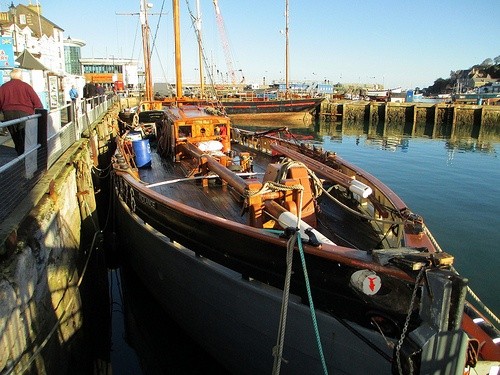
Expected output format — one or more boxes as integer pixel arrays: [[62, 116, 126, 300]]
[[133, 140, 152, 170]]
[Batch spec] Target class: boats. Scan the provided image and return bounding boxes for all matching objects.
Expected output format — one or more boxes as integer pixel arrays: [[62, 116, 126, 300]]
[[161, 0, 329, 122]]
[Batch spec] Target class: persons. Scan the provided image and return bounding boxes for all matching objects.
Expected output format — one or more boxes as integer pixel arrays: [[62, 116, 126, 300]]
[[69, 85, 78, 109], [83, 83, 105, 109], [0, 68, 44, 155]]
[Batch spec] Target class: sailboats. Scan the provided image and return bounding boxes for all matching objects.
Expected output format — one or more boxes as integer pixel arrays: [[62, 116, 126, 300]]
[[110, 0, 500, 374]]
